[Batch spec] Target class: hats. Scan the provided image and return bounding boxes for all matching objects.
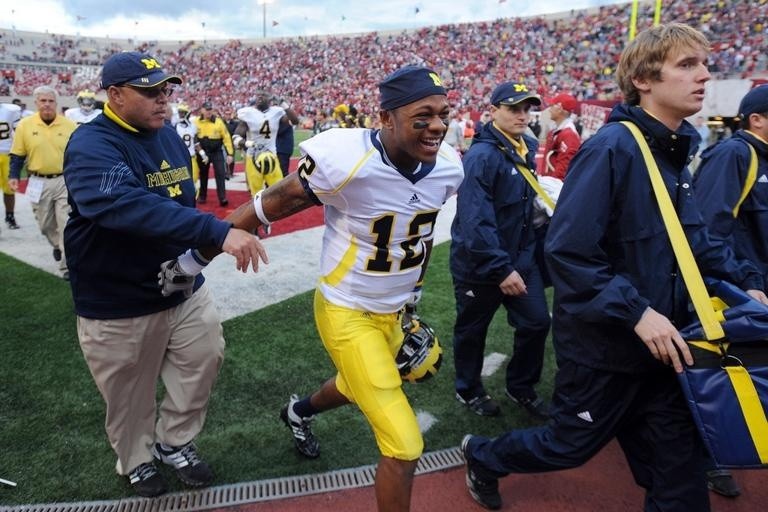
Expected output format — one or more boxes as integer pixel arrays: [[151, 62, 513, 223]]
[[737, 84, 767, 123], [202, 101, 213, 110], [376, 64, 448, 112], [99, 52, 183, 88], [489, 81, 542, 106], [543, 93, 577, 113]]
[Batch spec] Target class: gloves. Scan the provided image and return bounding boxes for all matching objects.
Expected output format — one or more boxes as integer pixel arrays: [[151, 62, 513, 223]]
[[198, 149, 210, 165], [157, 257, 196, 300]]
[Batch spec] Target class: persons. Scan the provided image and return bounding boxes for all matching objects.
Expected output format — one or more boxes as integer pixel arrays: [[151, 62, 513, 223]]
[[461, 24, 768, 512], [266, 95, 294, 177], [8, 86, 81, 280], [63, 51, 269, 496], [171, 105, 208, 201], [232, 92, 300, 235], [450, 82, 552, 419], [692, 83, 768, 498], [687, 117, 710, 177], [193, 103, 233, 206], [157, 64, 465, 512], [541, 94, 581, 180], [0, 102, 23, 230], [64, 90, 102, 123]]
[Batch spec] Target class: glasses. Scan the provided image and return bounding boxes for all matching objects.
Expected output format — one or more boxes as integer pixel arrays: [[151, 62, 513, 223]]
[[79, 102, 92, 107], [117, 85, 173, 98]]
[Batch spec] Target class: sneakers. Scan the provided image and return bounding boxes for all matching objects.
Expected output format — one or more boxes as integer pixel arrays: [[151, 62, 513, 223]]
[[504, 386, 552, 422], [460, 433, 504, 510], [454, 389, 502, 417], [151, 440, 212, 486], [125, 460, 169, 499], [279, 393, 322, 459], [5, 212, 20, 230], [704, 465, 744, 498]]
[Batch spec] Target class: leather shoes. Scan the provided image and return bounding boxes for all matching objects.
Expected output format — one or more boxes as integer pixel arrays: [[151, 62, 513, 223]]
[[199, 196, 206, 204], [53, 247, 63, 261], [62, 271, 71, 281], [220, 199, 228, 208]]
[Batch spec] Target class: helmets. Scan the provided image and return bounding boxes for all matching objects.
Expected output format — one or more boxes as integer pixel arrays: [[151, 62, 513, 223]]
[[75, 88, 96, 102], [251, 142, 276, 175], [393, 318, 444, 385]]
